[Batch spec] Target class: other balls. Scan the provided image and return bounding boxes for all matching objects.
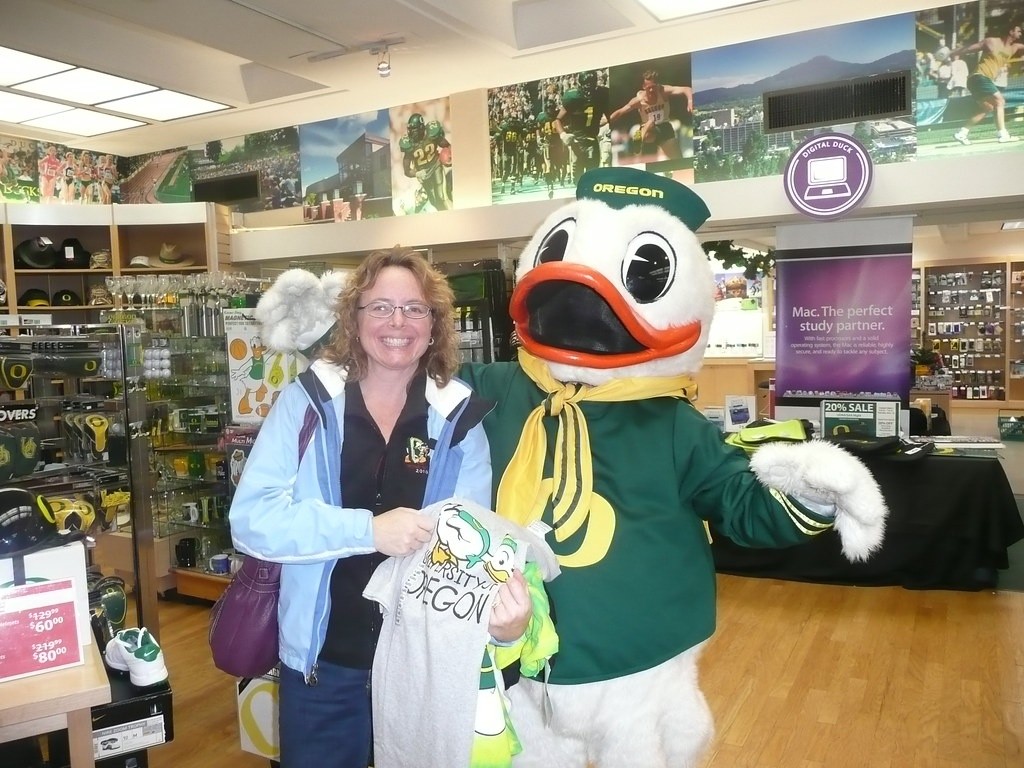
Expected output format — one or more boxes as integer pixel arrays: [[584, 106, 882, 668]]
[[142, 348, 171, 380], [97, 348, 122, 379]]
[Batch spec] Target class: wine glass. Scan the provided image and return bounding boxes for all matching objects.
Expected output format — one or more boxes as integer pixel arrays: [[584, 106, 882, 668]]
[[105, 270, 250, 313]]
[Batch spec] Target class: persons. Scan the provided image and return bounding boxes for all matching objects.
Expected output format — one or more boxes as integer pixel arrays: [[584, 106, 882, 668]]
[[399, 113, 452, 211], [488, 66, 610, 199], [916, 23, 1024, 145], [38, 141, 117, 204], [192, 149, 301, 211], [229, 245, 531, 768], [610, 71, 693, 160], [0, 140, 37, 204]]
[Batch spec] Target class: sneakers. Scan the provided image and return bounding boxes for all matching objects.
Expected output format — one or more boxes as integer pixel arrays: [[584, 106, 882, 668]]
[[998, 134, 1017, 142], [106, 626, 169, 686], [954, 132, 971, 145]]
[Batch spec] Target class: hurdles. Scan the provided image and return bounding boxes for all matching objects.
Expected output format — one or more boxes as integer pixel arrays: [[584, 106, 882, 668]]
[[624, 157, 694, 173]]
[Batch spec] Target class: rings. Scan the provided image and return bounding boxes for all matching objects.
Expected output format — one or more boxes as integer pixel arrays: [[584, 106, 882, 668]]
[[492, 600, 502, 608]]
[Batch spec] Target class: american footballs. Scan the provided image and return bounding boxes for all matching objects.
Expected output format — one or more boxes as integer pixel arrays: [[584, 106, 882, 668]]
[[439, 146, 451, 165]]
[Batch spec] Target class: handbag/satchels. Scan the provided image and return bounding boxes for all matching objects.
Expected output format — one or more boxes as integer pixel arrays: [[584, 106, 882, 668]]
[[207, 556, 283, 678]]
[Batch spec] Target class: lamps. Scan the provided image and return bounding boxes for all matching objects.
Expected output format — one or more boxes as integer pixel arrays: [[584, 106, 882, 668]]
[[367, 42, 394, 76]]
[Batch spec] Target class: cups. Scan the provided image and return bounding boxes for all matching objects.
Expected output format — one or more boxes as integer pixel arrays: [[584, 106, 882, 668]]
[[180, 493, 230, 529], [174, 534, 244, 577]]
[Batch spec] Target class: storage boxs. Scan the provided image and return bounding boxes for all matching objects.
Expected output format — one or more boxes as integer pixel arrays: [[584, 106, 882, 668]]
[[91, 678, 175, 762]]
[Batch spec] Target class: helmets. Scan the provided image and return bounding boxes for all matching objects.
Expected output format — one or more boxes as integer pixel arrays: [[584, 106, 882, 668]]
[[545, 100, 557, 114], [578, 71, 598, 97], [0, 488, 59, 559], [406, 113, 424, 141]]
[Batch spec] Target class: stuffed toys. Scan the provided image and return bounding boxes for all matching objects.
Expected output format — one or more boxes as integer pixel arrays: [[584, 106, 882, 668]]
[[256, 164, 890, 768]]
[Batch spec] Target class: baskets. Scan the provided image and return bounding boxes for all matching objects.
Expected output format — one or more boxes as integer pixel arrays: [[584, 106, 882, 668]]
[[998, 416, 1024, 442]]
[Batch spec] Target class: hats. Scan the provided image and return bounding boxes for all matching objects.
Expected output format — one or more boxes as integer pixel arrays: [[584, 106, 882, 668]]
[[52, 289, 82, 306], [90, 250, 112, 268], [88, 283, 114, 306], [21, 289, 50, 308], [18, 238, 56, 268], [128, 256, 151, 268], [149, 242, 196, 267], [56, 237, 89, 269]]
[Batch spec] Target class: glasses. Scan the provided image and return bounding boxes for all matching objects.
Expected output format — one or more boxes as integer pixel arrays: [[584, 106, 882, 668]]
[[356, 301, 434, 319]]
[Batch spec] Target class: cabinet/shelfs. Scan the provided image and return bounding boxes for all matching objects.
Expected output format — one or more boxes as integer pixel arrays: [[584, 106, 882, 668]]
[[15, 268, 114, 310], [134, 325, 237, 600]]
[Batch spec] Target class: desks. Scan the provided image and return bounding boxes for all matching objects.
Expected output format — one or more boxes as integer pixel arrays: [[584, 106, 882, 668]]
[[710, 431, 1023, 591]]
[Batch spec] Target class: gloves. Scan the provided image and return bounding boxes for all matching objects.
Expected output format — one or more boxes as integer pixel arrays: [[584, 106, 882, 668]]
[[417, 167, 429, 183], [561, 132, 578, 147]]
